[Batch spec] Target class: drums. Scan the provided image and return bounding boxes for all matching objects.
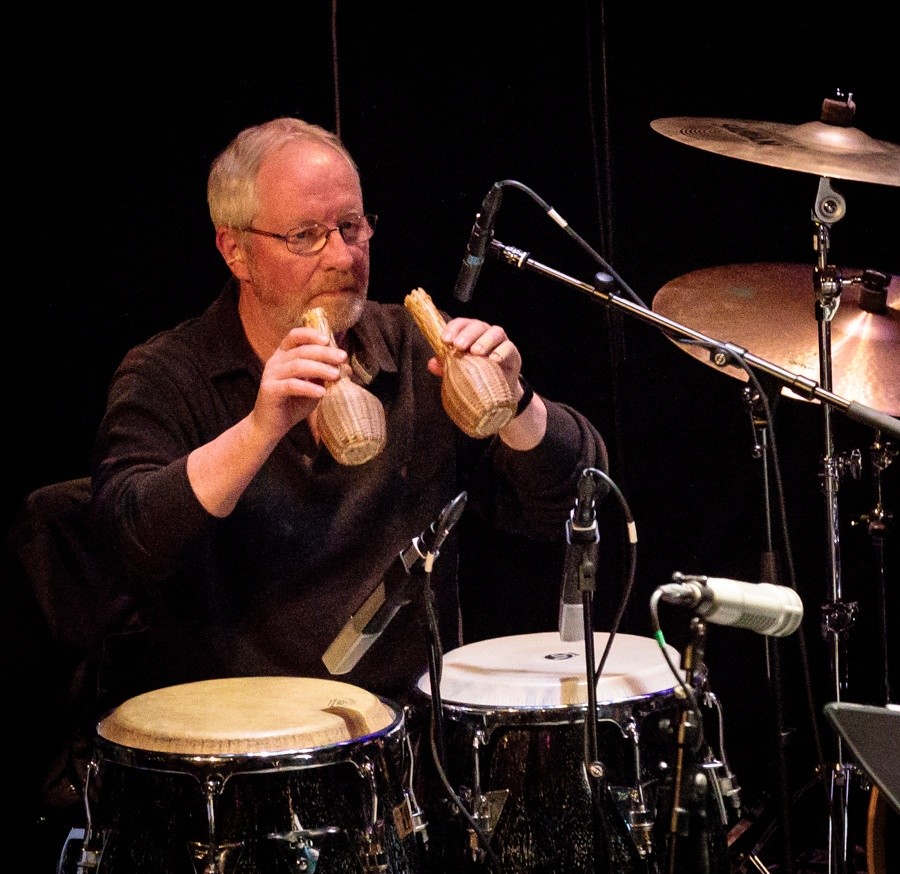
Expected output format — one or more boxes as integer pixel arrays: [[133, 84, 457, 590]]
[[413, 631, 712, 874], [90, 676, 421, 874]]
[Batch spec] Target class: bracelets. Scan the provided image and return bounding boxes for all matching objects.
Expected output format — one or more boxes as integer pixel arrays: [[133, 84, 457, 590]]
[[514, 372, 533, 418]]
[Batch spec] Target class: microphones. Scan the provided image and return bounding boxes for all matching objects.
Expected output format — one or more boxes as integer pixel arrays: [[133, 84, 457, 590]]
[[323, 495, 468, 675], [659, 577, 804, 638], [559, 477, 600, 641], [454, 181, 504, 302]]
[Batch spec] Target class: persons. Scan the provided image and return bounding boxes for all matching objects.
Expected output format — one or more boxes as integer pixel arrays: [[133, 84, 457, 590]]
[[96, 118, 611, 720]]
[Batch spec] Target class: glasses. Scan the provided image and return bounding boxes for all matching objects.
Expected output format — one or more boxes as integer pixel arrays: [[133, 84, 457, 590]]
[[244, 214, 374, 256]]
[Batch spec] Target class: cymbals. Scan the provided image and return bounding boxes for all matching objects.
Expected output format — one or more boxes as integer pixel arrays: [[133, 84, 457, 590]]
[[652, 264, 900, 417], [649, 118, 900, 186]]
